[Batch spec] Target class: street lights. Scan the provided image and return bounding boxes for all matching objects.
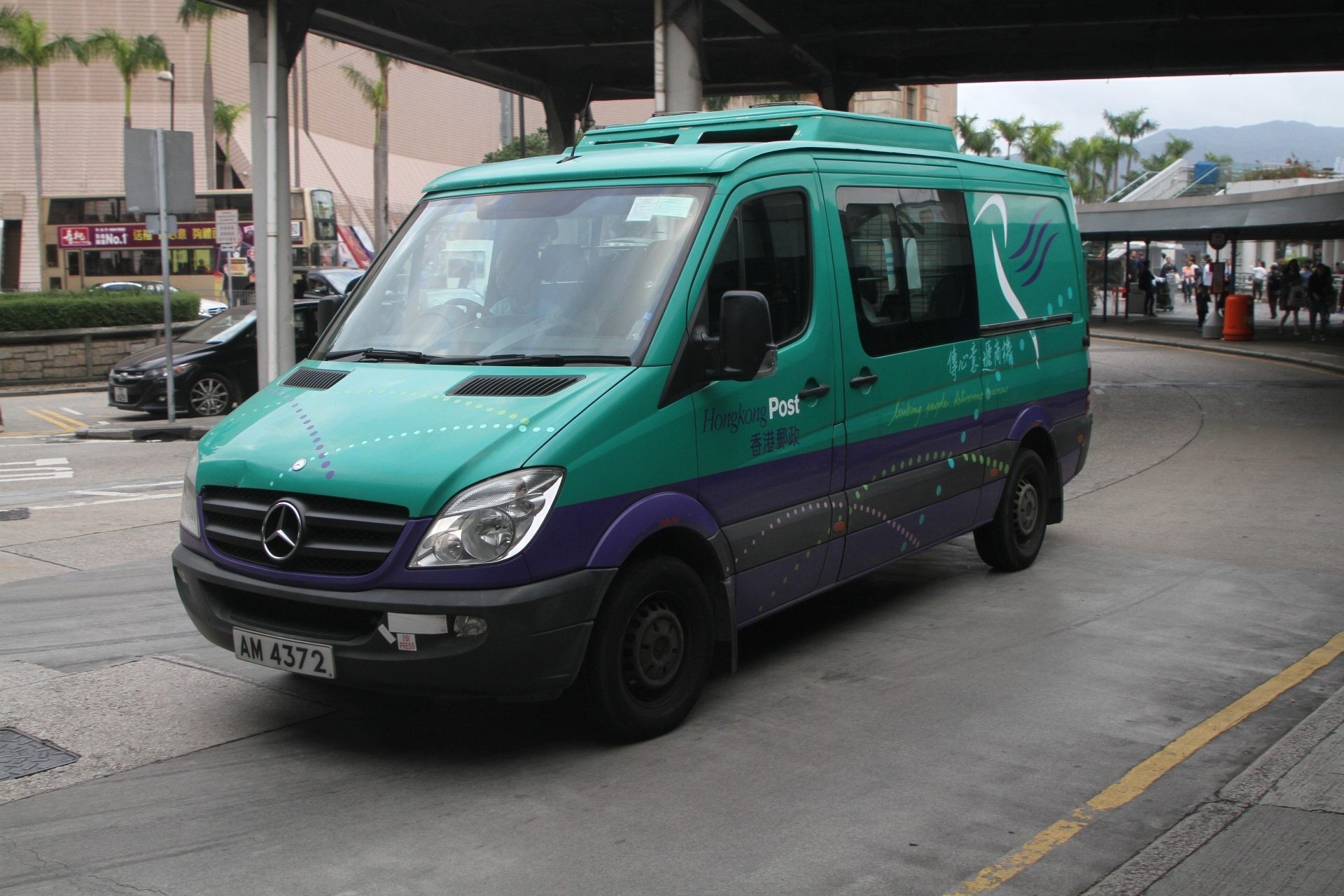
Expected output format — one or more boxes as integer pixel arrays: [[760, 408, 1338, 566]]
[[157, 63, 176, 131]]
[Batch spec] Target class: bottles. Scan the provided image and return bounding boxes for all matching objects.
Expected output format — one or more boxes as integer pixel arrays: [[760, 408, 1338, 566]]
[[626, 313, 652, 340]]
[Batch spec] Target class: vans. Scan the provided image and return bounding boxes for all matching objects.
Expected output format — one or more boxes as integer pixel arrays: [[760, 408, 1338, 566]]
[[170, 99, 1096, 744]]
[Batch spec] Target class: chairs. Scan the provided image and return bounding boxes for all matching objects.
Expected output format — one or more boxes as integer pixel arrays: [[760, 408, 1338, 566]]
[[535, 243, 613, 336]]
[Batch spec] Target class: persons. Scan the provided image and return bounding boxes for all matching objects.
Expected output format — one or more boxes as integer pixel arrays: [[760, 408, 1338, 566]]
[[1264, 263, 1279, 318], [478, 234, 564, 331], [1161, 254, 1180, 308], [1139, 258, 1156, 317], [1203, 253, 1214, 303], [219, 225, 255, 308], [1135, 251, 1146, 281], [1300, 262, 1311, 311], [1278, 257, 1303, 338], [1248, 260, 1266, 300], [1217, 257, 1231, 316], [1190, 282, 1212, 328], [1127, 250, 1137, 295], [1307, 263, 1336, 342], [1181, 255, 1202, 302]]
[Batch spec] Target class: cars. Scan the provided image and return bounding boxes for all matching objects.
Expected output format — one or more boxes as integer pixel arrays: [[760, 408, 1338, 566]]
[[292, 266, 367, 299], [88, 279, 228, 318], [106, 299, 321, 419]]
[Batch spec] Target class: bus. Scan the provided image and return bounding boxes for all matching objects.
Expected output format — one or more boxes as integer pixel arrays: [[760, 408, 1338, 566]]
[[39, 186, 341, 303]]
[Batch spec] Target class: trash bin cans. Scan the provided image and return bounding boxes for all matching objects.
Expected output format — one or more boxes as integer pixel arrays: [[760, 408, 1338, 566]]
[[1221, 294, 1253, 341]]
